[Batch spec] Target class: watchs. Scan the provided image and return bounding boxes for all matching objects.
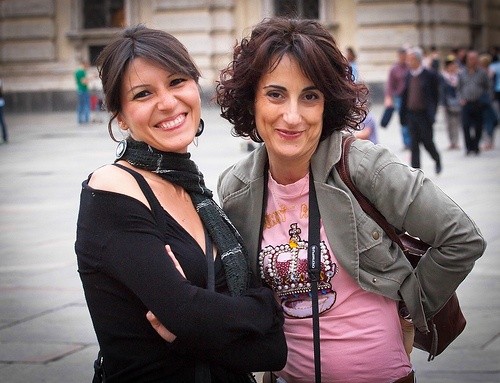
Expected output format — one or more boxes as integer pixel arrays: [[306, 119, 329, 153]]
[[398, 303, 413, 323]]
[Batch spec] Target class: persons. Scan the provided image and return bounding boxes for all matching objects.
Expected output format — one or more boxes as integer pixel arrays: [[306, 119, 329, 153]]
[[399, 45, 446, 174], [438, 47, 500, 157], [74, 25, 288, 383], [344, 43, 361, 85], [74, 61, 97, 124], [348, 110, 378, 145], [0, 84, 8, 144], [385, 45, 414, 151], [216, 15, 487, 383]]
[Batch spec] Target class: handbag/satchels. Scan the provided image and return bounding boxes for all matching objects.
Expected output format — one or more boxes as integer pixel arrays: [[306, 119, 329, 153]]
[[335, 135, 467, 361]]
